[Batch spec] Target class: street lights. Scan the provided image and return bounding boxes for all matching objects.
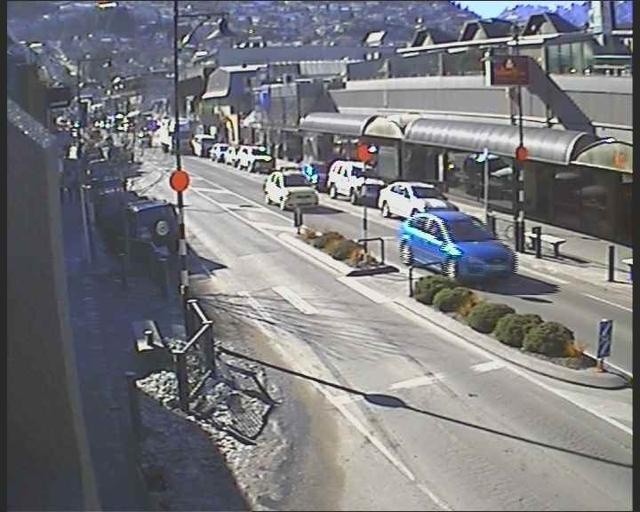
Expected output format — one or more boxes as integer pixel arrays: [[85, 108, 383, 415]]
[[505, 24, 528, 253], [172, 10, 237, 208], [75, 58, 112, 104]]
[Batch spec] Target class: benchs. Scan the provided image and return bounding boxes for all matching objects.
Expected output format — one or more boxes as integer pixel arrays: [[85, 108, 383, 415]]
[[525, 232, 566, 258], [621, 258, 633, 283]]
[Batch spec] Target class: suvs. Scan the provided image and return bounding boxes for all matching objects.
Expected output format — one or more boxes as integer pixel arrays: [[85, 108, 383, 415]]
[[234, 143, 273, 174]]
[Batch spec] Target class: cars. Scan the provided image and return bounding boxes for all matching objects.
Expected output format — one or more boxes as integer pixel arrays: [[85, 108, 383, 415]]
[[262, 170, 318, 210], [398, 210, 516, 286], [94, 108, 238, 168], [304, 157, 459, 214]]
